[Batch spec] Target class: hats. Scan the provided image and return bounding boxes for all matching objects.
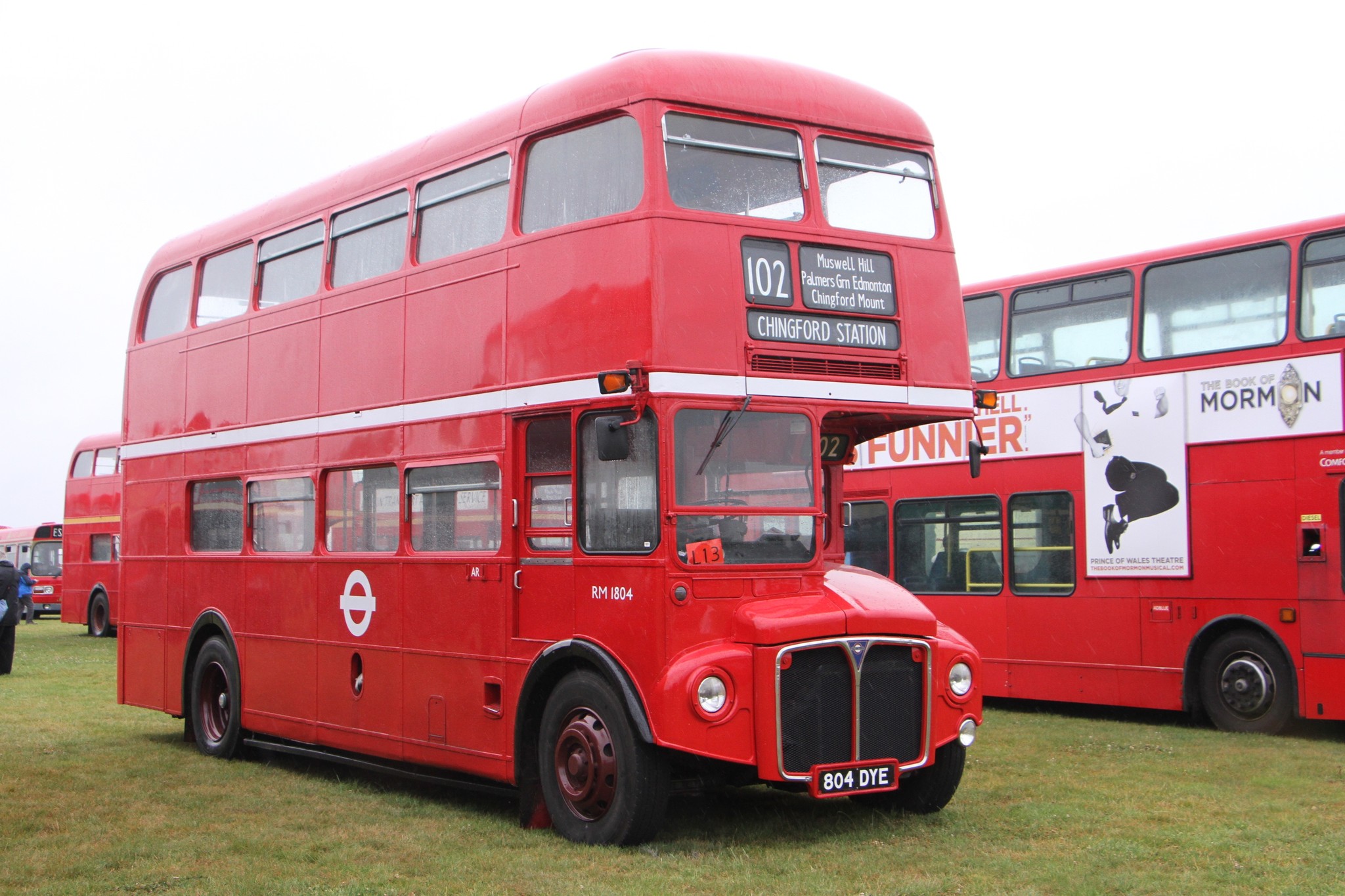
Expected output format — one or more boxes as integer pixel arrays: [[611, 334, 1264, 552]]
[[21, 563, 31, 570]]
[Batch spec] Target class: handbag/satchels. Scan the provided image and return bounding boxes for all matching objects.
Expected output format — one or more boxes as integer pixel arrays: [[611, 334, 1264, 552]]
[[0, 567, 15, 621]]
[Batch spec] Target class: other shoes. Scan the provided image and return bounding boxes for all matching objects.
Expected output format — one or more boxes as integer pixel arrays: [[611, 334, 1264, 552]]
[[17, 621, 21, 624], [26, 621, 37, 625]]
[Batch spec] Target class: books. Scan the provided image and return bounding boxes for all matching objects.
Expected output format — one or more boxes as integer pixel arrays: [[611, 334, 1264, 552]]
[[1091, 429, 1113, 457]]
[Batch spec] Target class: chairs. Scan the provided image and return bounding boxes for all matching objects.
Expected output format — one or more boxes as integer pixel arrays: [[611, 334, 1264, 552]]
[[589, 509, 656, 550], [927, 550, 1003, 591], [990, 368, 999, 379], [1019, 357, 1045, 376], [1047, 359, 1074, 372], [970, 357, 990, 381]]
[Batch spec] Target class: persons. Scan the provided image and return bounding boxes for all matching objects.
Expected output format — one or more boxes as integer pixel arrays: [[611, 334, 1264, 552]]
[[1074, 377, 1180, 554], [0, 550, 20, 675], [18, 563, 38, 625]]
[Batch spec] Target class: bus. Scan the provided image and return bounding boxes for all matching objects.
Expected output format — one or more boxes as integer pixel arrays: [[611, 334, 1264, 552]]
[[0, 525, 63, 619], [57, 437, 120, 641], [659, 210, 1345, 733], [113, 43, 989, 849]]
